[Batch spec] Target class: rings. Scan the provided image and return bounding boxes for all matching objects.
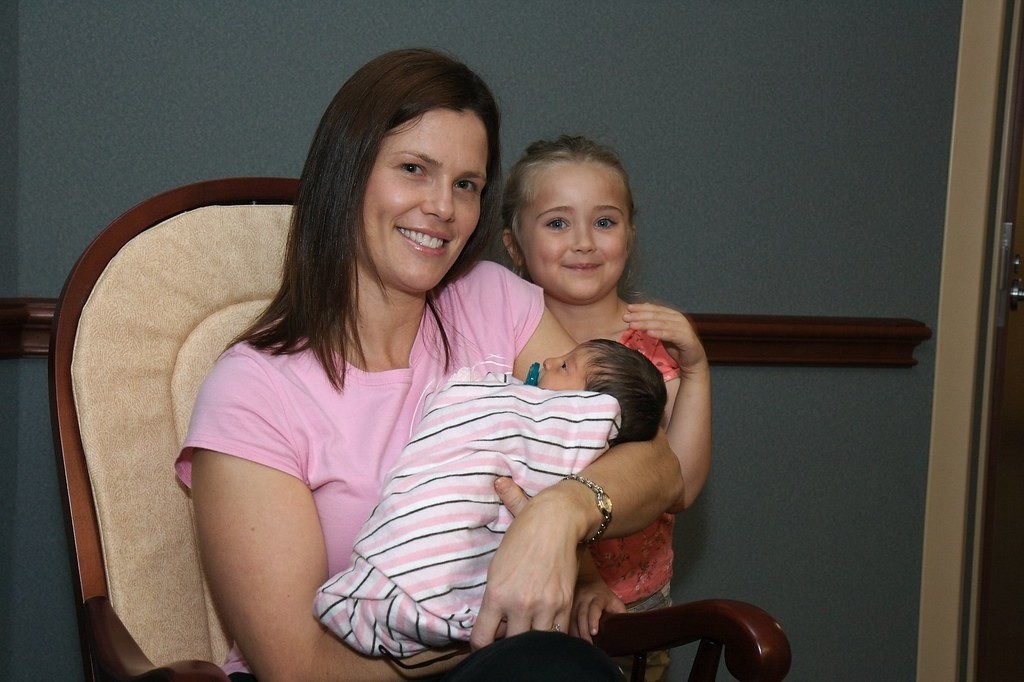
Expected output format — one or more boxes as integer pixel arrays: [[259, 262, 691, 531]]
[[551, 623, 561, 631]]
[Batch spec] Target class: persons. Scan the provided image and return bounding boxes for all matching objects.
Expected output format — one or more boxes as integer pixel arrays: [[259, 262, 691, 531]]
[[314, 338, 670, 657], [175, 47, 685, 682], [498, 134, 711, 682]]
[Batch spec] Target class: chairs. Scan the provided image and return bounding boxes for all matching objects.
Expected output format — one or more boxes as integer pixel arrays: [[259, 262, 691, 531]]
[[49, 177, 791, 682]]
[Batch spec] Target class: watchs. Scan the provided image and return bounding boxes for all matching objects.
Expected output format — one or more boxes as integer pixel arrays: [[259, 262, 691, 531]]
[[561, 474, 613, 544]]
